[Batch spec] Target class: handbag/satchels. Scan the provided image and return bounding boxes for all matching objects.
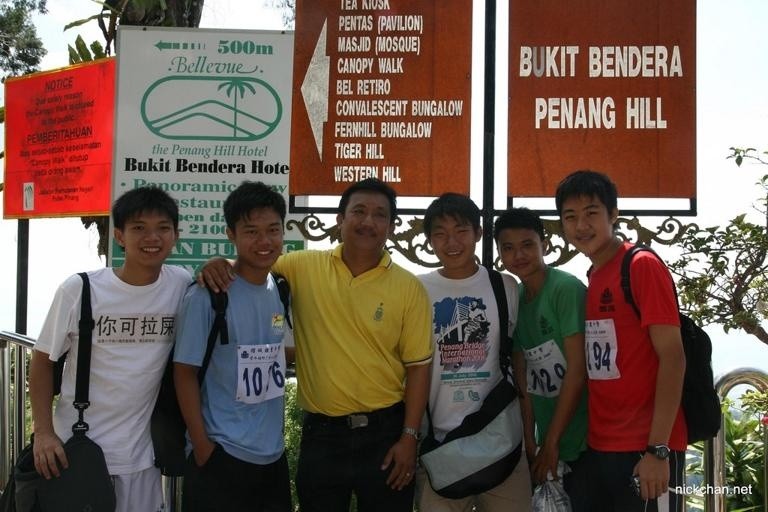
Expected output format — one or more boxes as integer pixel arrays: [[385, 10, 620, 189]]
[[420, 377, 522, 498], [0, 430, 116, 512]]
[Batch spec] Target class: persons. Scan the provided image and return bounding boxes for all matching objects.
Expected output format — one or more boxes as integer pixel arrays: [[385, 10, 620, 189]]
[[551, 168, 685, 512], [174, 179, 294, 512], [414, 193, 534, 512], [29, 187, 191, 511], [195, 174, 435, 512], [495, 204, 587, 512]]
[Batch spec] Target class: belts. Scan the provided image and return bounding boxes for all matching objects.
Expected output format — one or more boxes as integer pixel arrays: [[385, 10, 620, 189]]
[[304, 400, 403, 428]]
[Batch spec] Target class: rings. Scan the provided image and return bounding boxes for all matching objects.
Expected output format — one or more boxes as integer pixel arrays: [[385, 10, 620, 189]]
[[405, 472, 413, 478]]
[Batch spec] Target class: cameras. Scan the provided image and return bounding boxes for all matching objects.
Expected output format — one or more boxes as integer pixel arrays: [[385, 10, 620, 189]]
[[629, 475, 640, 497]]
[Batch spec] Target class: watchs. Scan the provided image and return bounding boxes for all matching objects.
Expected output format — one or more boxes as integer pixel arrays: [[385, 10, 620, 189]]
[[645, 442, 670, 461], [399, 426, 422, 442]]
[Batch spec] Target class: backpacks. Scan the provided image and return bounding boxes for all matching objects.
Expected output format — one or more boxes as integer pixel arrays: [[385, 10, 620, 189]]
[[621, 245, 721, 442], [150, 272, 292, 476]]
